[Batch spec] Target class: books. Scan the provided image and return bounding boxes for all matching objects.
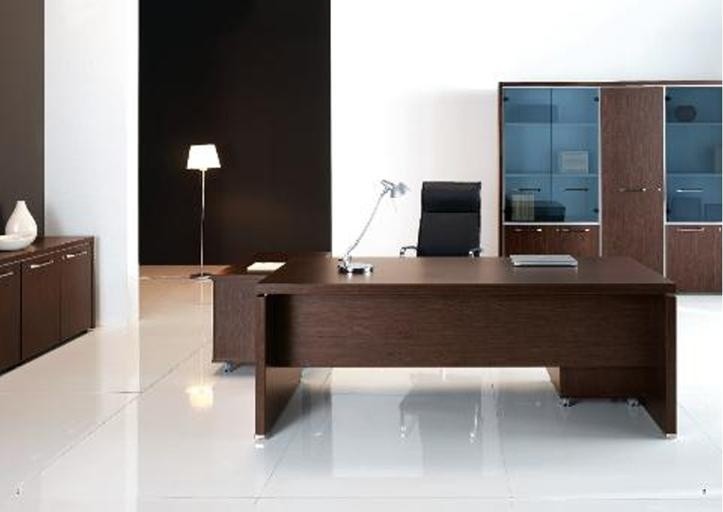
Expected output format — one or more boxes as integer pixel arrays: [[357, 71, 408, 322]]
[[510, 193, 535, 221]]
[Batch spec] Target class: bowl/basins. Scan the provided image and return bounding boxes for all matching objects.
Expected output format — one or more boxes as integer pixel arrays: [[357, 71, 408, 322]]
[[0, 234, 32, 251]]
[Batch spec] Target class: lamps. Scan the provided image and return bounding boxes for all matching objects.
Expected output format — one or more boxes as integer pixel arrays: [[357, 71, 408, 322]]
[[335, 178, 408, 275], [186, 142, 221, 282]]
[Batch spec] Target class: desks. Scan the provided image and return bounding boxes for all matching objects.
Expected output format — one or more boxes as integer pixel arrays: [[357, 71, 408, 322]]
[[252, 254, 678, 443]]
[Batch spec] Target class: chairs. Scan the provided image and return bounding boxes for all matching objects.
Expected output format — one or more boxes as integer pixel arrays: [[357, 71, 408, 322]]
[[394, 368, 489, 487], [398, 181, 482, 257]]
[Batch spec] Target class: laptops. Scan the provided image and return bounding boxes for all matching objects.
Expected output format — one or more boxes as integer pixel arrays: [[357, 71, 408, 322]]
[[510, 254, 578, 267]]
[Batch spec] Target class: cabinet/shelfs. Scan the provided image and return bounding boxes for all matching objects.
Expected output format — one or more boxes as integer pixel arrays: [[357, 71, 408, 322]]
[[599, 81, 662, 270], [498, 80, 599, 257], [544, 365, 641, 408], [206, 265, 272, 374], [20, 251, 61, 365], [61, 242, 91, 344], [662, 80, 722, 293], [1, 262, 22, 375]]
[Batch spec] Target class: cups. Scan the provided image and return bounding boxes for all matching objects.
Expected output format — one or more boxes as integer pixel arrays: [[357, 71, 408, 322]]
[[674, 104, 696, 121]]
